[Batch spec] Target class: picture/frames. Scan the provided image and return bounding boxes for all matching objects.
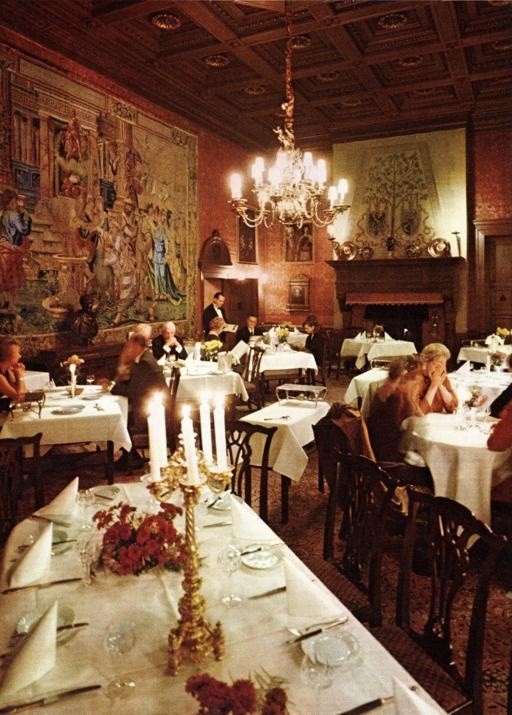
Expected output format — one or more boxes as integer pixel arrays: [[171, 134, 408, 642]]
[[281, 223, 316, 313], [236, 212, 260, 267]]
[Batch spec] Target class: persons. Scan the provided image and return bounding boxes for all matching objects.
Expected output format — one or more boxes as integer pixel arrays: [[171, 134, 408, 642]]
[[152, 320, 188, 360], [202, 292, 230, 344], [294, 319, 329, 383], [206, 317, 227, 343], [68, 295, 99, 339], [236, 313, 263, 342], [410, 342, 458, 415], [284, 226, 312, 260], [116, 324, 152, 381], [1, 339, 29, 414], [110, 332, 172, 476], [366, 354, 423, 488], [292, 288, 305, 304]]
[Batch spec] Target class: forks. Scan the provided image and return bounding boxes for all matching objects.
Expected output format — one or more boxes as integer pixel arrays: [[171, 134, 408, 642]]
[[287, 618, 342, 635]]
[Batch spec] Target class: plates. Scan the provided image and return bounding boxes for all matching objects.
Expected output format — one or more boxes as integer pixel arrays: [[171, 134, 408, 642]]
[[24, 529, 69, 555], [207, 495, 247, 511], [14, 604, 77, 644], [55, 404, 85, 414], [302, 629, 361, 664], [88, 485, 122, 503], [240, 544, 285, 570]]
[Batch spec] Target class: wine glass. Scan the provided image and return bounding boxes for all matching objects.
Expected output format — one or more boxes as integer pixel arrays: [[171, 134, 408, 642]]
[[216, 546, 244, 610], [92, 616, 137, 700], [300, 653, 337, 715], [451, 403, 492, 434], [75, 486, 95, 528], [458, 361, 512, 387]]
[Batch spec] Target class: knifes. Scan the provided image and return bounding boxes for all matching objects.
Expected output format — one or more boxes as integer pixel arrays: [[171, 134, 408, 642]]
[[17, 539, 77, 550], [246, 580, 316, 600], [12, 623, 89, 639], [282, 617, 348, 646], [207, 489, 232, 508]]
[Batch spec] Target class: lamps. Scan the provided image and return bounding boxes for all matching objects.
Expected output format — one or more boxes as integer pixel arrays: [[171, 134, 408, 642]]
[[227, 0, 351, 232]]
